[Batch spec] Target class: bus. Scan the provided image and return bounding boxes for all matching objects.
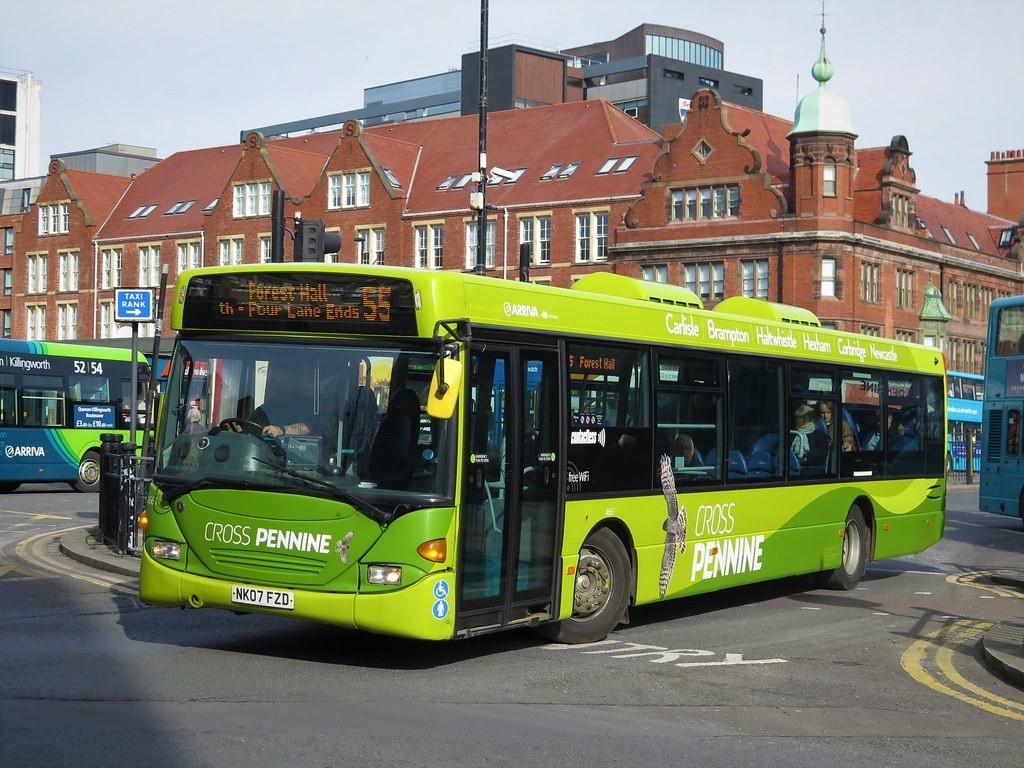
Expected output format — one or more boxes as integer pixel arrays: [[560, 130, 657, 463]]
[[0, 337, 153, 494], [944, 369, 985, 473], [976, 292, 1024, 521], [130, 251, 954, 646]]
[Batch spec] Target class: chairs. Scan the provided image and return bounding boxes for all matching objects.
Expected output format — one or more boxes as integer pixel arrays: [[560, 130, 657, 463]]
[[345, 385, 378, 467], [691, 403, 919, 480]]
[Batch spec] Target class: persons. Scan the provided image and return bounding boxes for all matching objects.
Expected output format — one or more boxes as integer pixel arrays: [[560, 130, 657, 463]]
[[669, 431, 708, 486], [773, 398, 904, 468], [217, 365, 316, 466]]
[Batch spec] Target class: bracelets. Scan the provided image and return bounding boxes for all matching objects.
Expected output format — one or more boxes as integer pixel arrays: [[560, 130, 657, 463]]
[[280, 426, 286, 434]]
